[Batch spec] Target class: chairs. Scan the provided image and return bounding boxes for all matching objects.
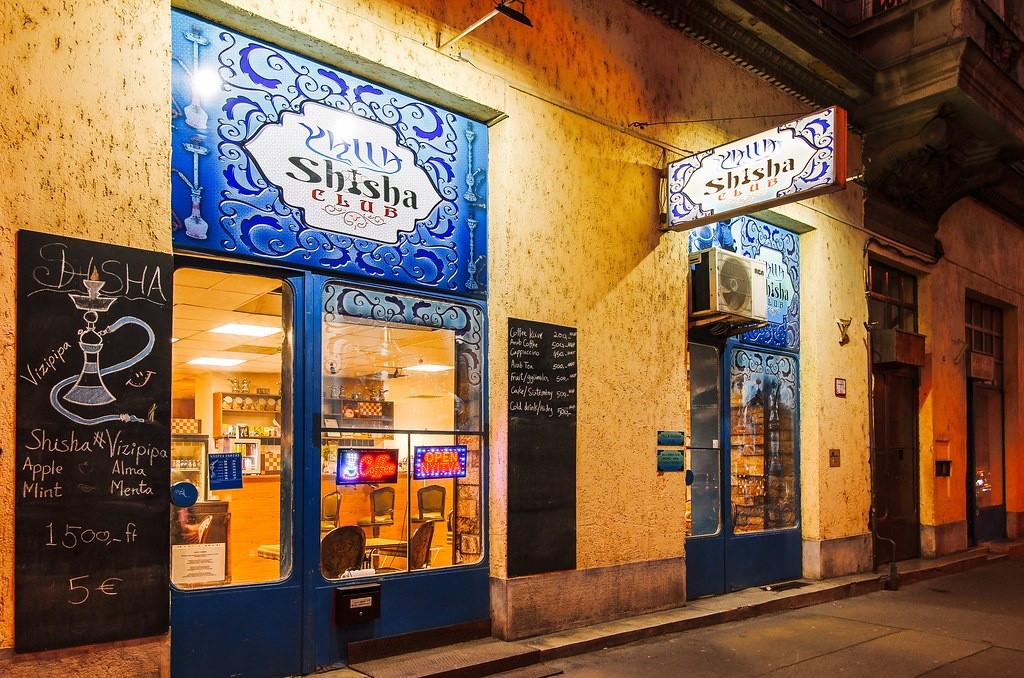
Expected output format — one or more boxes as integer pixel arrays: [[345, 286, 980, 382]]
[[409, 519, 435, 570], [357, 486, 395, 536], [320, 491, 341, 533], [412, 485, 448, 525]]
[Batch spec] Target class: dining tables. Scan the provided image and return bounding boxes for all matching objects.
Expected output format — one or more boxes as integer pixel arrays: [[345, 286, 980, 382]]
[[362, 535, 405, 576]]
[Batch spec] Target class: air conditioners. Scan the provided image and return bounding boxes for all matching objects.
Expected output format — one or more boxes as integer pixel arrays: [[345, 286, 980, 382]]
[[691, 250, 776, 336]]
